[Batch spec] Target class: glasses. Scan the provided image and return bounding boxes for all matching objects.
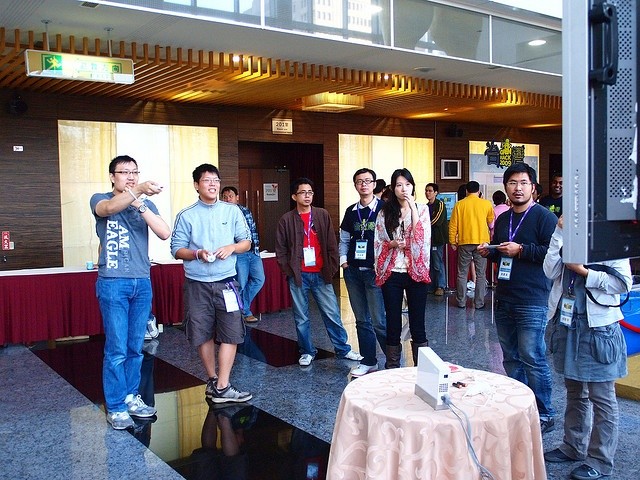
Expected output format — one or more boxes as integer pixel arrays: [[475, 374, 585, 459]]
[[355, 178, 375, 185], [507, 182, 532, 187], [201, 179, 221, 183], [114, 170, 140, 176], [295, 191, 314, 196]]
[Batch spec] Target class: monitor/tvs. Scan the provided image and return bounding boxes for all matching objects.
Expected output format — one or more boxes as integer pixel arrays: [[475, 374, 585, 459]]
[[560, 0, 640, 268]]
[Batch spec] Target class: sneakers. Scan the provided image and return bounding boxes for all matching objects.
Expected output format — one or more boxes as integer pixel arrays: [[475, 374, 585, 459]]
[[145, 332, 152, 340], [545, 448, 575, 462], [212, 383, 252, 403], [205, 377, 218, 399], [244, 316, 258, 323], [107, 411, 134, 430], [467, 280, 476, 290], [571, 464, 603, 480], [127, 395, 157, 417], [350, 364, 378, 377], [148, 317, 159, 339], [299, 354, 313, 366], [435, 288, 444, 295], [345, 350, 365, 361], [540, 418, 554, 436]]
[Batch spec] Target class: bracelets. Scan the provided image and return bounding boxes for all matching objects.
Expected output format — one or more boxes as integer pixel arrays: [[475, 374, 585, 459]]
[[518, 244, 523, 259]]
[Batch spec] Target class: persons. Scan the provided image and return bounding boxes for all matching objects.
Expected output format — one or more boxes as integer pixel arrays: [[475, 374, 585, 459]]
[[373, 179, 393, 202], [532, 184, 542, 203], [489, 190, 510, 243], [540, 173, 563, 218], [89, 154, 171, 429], [96, 223, 159, 341], [467, 192, 489, 290], [542, 214, 634, 480], [449, 181, 494, 310], [373, 167, 432, 369], [477, 162, 559, 437], [425, 183, 447, 296], [222, 186, 266, 323], [338, 168, 386, 377], [171, 163, 253, 404], [274, 177, 364, 366]]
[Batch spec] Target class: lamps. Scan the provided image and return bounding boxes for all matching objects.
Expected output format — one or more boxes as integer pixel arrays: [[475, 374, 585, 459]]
[[302, 91, 365, 113]]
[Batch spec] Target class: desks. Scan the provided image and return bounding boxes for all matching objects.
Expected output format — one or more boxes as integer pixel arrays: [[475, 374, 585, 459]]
[[325, 366, 547, 480], [0, 254, 292, 348]]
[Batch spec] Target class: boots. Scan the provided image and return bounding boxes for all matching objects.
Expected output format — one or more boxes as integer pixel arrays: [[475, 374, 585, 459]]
[[410, 340, 428, 367], [385, 344, 402, 369]]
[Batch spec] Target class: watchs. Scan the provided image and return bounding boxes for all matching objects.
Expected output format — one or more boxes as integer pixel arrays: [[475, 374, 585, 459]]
[[138, 204, 146, 214]]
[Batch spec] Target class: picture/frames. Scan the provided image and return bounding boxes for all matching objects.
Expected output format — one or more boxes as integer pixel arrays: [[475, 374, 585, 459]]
[[441, 159, 462, 180], [436, 192, 458, 220]]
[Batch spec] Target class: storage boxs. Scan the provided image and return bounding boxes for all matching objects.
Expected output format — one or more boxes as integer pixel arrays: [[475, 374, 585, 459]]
[[620, 291, 640, 356]]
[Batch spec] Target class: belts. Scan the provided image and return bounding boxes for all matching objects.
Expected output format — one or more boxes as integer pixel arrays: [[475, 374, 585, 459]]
[[188, 277, 233, 283]]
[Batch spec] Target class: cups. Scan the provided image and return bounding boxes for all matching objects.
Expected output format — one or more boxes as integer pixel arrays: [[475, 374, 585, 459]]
[[86, 261, 93, 270]]
